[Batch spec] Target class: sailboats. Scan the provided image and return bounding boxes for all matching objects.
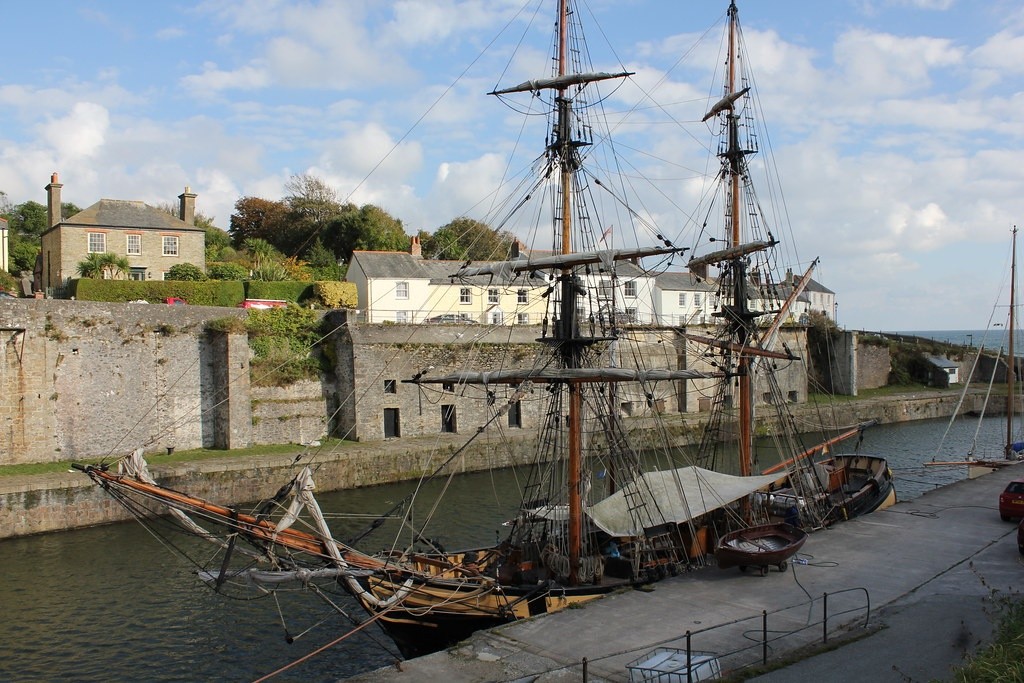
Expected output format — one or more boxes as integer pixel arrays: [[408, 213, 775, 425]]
[[919, 224, 1023, 482], [71, 0, 900, 663]]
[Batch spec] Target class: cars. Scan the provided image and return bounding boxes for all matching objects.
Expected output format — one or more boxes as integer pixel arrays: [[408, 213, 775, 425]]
[[999, 480, 1024, 521]]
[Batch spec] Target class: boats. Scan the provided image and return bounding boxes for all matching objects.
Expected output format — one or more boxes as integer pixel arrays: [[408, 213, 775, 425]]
[[716, 523, 809, 568]]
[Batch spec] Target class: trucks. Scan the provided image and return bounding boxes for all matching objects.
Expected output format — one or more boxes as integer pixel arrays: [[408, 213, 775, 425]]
[[238, 298, 288, 309], [122, 296, 189, 306]]
